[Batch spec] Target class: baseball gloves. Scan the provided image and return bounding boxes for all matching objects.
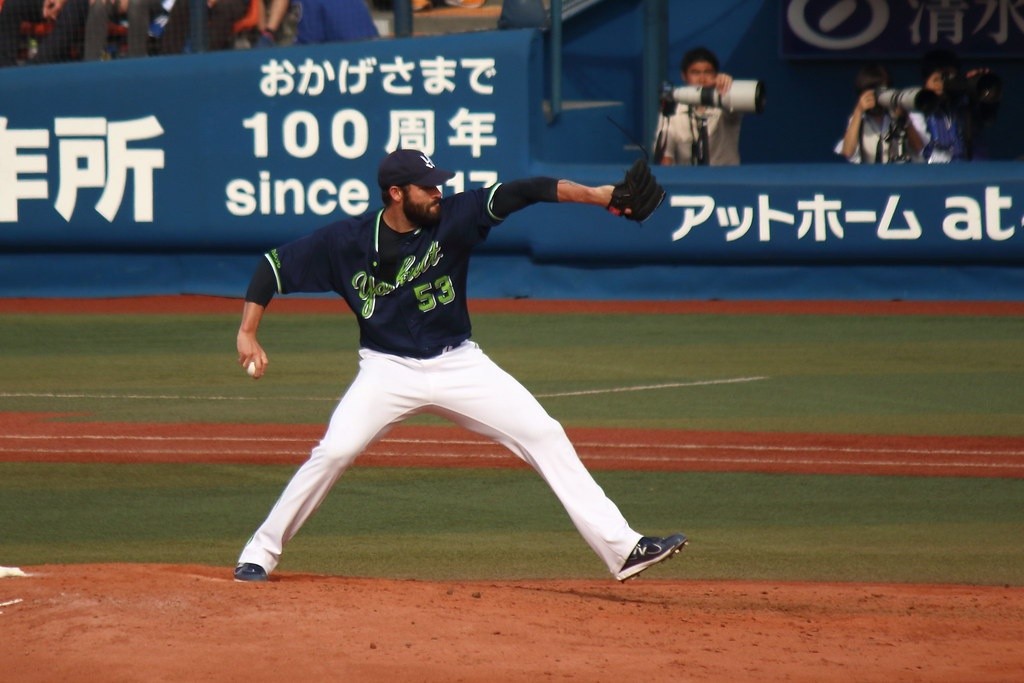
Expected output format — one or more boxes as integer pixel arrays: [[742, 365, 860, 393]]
[[609, 158, 665, 224]]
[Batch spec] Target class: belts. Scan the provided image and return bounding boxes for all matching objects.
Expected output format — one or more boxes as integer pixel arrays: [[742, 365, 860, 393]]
[[417, 342, 461, 358]]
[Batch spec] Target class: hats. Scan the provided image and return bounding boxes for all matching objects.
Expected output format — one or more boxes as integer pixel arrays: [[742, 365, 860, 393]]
[[379, 149, 456, 191]]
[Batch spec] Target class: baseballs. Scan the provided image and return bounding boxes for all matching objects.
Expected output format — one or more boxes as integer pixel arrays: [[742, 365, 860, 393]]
[[246, 361, 256, 378]]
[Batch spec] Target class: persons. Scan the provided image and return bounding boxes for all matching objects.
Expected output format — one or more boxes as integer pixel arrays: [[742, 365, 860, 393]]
[[654, 48, 743, 165], [232, 150, 687, 584], [835, 50, 990, 163], [1, 0, 290, 66]]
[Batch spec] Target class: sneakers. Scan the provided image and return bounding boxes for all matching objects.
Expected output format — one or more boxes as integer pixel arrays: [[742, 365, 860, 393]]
[[234, 562, 268, 583], [617, 534, 689, 584]]
